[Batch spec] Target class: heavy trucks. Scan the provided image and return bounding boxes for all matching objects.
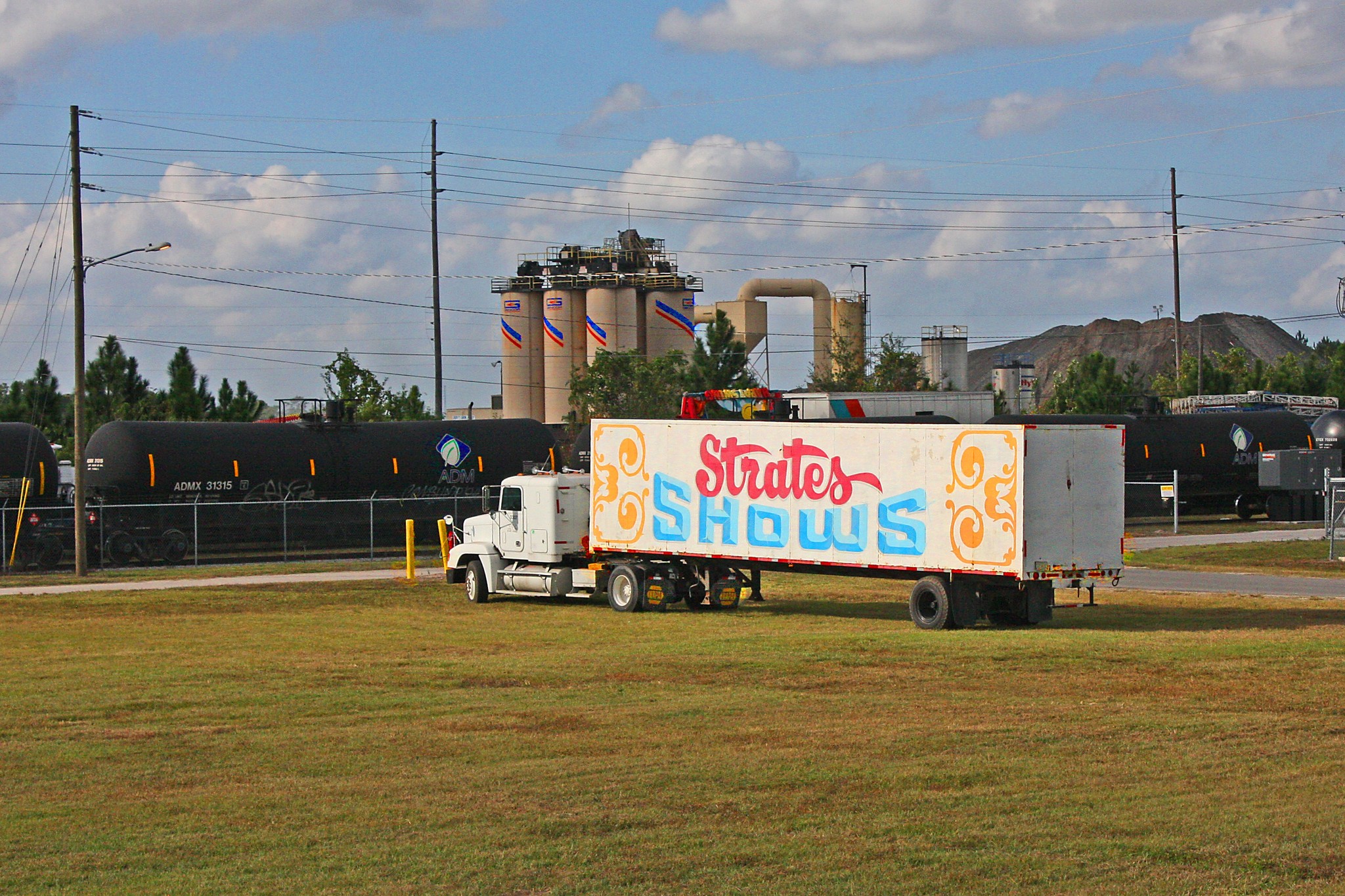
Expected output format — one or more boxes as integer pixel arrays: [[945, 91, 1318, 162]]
[[445, 415, 1127, 631]]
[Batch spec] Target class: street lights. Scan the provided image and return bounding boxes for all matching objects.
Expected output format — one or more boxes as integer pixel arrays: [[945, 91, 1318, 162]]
[[74, 241, 171, 573]]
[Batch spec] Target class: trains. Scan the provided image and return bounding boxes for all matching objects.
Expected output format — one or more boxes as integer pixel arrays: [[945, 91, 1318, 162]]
[[0, 408, 1345, 564]]
